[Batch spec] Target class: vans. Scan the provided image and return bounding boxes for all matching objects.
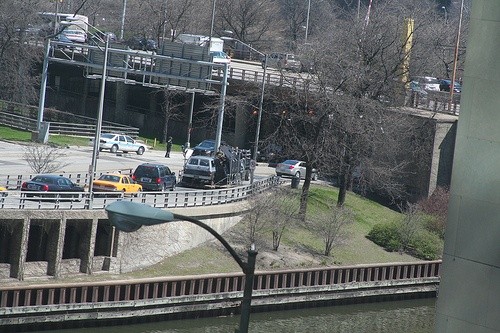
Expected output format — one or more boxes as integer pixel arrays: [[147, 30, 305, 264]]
[[262, 52, 302, 72]]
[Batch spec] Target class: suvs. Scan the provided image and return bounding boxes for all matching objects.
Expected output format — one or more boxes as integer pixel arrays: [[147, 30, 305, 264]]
[[131, 163, 177, 191]]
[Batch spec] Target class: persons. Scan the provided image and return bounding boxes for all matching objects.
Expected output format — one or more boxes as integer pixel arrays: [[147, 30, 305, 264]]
[[181, 144, 189, 159], [165, 137, 172, 158]]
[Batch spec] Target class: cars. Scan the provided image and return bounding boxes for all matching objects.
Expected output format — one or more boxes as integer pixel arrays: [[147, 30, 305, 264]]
[[128, 39, 158, 51], [403, 76, 441, 92], [20, 175, 85, 202], [440, 79, 462, 93], [96, 30, 116, 41], [209, 50, 232, 70], [0, 187, 8, 202], [92, 173, 142, 197], [181, 140, 251, 183], [89, 133, 148, 155], [276, 159, 319, 181]]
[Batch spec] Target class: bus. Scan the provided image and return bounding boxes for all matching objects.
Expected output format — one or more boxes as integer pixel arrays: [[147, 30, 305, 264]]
[[35, 12, 89, 44]]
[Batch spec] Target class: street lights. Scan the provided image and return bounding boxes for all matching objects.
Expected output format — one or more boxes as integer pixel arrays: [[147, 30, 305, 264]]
[[59, 17, 112, 204], [219, 36, 268, 186]]
